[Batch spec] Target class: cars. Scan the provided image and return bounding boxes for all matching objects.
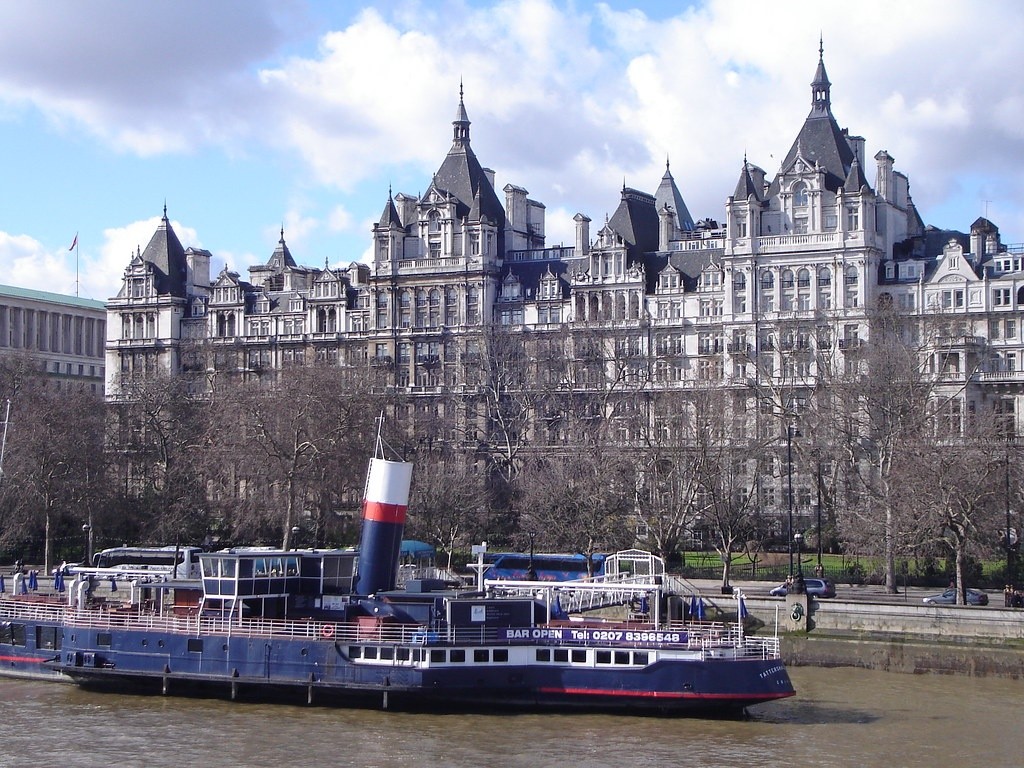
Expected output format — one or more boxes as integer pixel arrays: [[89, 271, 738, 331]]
[[51, 563, 83, 577], [922, 587, 990, 607], [769, 579, 836, 600]]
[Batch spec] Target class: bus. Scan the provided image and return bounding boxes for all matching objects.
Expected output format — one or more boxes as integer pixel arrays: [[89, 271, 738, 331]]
[[93, 546, 204, 579], [483, 552, 606, 587]]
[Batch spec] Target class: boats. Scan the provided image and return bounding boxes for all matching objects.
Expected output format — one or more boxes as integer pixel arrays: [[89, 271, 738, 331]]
[[0, 411, 796, 718]]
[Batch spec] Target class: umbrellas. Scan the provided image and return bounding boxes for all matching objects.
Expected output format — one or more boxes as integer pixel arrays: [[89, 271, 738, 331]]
[[552, 594, 563, 621], [1, 574, 5, 598], [21, 569, 39, 595], [111, 575, 118, 597], [639, 595, 648, 623], [53, 572, 65, 598], [162, 575, 169, 603], [689, 592, 706, 629], [736, 595, 749, 635]]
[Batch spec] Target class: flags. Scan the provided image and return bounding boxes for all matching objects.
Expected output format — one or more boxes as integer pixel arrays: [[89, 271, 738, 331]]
[[69, 236, 77, 251]]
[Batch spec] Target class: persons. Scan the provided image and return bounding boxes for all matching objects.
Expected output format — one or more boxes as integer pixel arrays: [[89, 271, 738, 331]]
[[949, 577, 954, 589]]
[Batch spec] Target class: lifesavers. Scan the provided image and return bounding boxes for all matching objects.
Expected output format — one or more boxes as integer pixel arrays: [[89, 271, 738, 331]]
[[789, 611, 801, 622], [322, 624, 334, 637]]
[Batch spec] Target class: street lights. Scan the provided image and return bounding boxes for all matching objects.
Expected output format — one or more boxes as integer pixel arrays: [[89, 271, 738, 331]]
[[81, 522, 91, 567], [526, 527, 539, 582], [794, 530, 806, 595], [291, 524, 301, 553]]
